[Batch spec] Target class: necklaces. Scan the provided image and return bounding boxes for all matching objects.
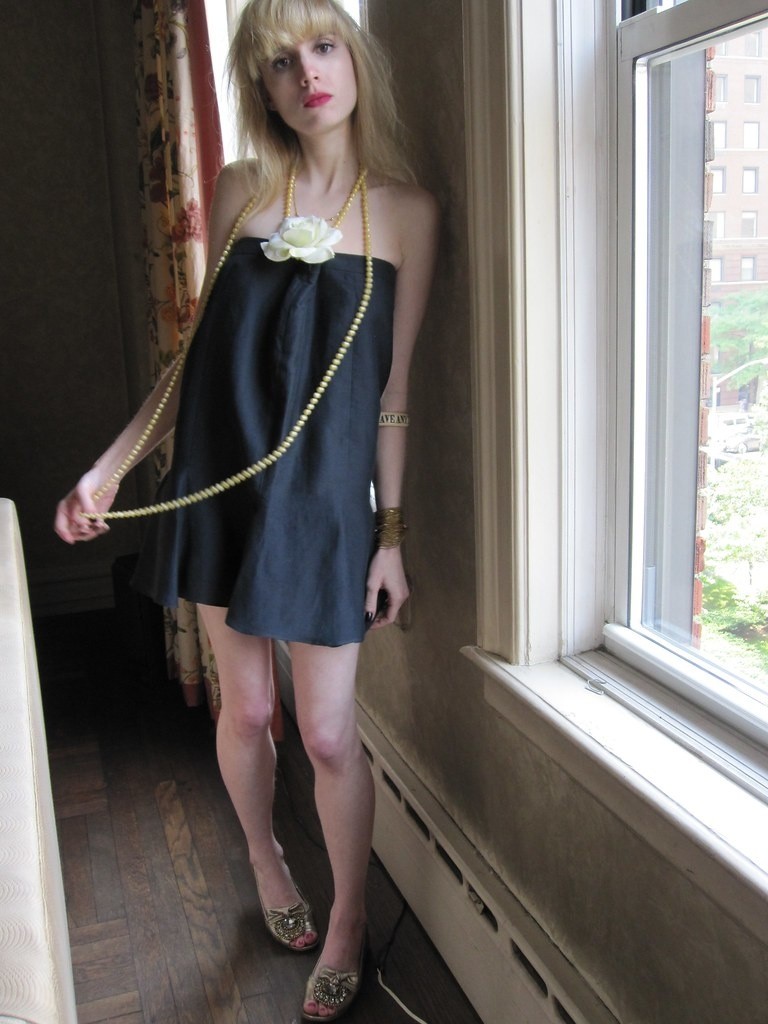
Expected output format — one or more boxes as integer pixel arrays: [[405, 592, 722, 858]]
[[77, 150, 374, 522]]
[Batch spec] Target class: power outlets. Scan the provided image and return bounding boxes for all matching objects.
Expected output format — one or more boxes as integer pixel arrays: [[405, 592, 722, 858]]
[[394, 584, 414, 632]]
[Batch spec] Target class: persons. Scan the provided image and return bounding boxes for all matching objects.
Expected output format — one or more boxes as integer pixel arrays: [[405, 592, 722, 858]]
[[53, 0, 441, 1023]]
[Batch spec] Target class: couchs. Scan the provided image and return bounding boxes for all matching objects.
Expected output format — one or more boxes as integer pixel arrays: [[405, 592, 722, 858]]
[[0, 495, 78, 1024]]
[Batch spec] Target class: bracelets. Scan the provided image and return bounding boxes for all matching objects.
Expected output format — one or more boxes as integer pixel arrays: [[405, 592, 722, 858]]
[[373, 508, 411, 550]]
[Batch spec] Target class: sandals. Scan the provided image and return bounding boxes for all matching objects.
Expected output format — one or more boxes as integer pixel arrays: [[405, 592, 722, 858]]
[[299, 932, 371, 1022], [253, 864, 320, 951]]
